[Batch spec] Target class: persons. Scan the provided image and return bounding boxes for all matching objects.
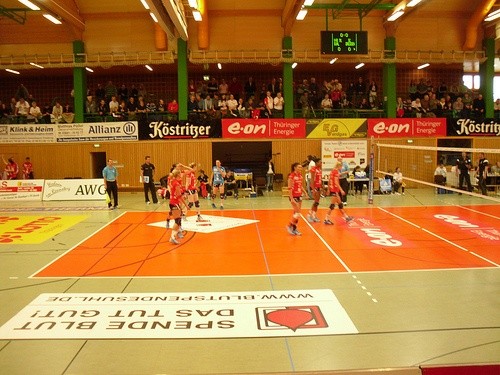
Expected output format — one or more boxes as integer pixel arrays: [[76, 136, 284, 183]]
[[333, 156, 350, 207], [395, 77, 486, 119], [323, 162, 354, 225], [2, 154, 34, 180], [306, 156, 322, 223], [434, 162, 447, 194], [197, 159, 239, 210], [86, 80, 179, 121], [188, 76, 284, 118], [352, 166, 406, 195], [457, 152, 474, 196], [140, 156, 161, 205], [296, 76, 378, 118], [166, 162, 204, 244], [474, 152, 491, 196], [494, 99, 500, 118], [102, 158, 121, 210], [287, 162, 310, 236], [264, 157, 276, 193], [0, 96, 75, 124], [304, 154, 324, 200]]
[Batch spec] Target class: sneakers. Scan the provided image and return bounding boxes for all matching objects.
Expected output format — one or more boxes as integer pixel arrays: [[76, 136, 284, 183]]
[[345, 217, 354, 223], [288, 227, 293, 234], [293, 230, 302, 236], [323, 219, 333, 225]]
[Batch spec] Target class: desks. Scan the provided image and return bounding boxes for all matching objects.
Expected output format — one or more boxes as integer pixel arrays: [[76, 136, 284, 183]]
[[321, 177, 370, 197], [476, 175, 500, 194]]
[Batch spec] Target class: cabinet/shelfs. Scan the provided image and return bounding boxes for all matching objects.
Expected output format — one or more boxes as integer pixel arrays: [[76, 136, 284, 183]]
[[234, 172, 254, 198]]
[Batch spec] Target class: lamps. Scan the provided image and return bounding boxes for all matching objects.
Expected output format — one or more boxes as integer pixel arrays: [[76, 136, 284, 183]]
[[18, 0, 62, 24], [386, 0, 422, 22], [295, 0, 314, 21], [188, 0, 203, 22], [483, 9, 500, 21], [140, 0, 158, 22]]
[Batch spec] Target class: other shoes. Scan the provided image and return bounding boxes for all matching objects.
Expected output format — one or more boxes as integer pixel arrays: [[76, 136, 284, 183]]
[[307, 213, 312, 222], [157, 201, 160, 204], [114, 205, 120, 209], [178, 232, 184, 238], [108, 206, 114, 209], [212, 203, 216, 208], [343, 201, 347, 205], [169, 238, 180, 244], [221, 206, 224, 210], [166, 219, 169, 227], [198, 216, 203, 221], [313, 218, 321, 222], [146, 201, 150, 204]]
